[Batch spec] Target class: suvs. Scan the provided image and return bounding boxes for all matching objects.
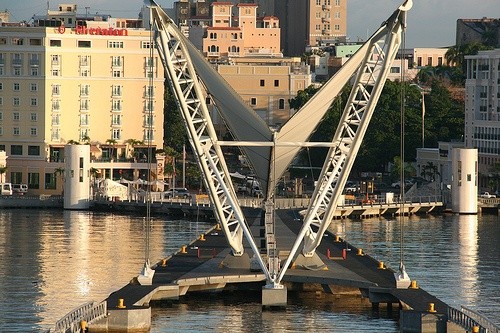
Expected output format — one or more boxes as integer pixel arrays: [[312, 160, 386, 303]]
[[163, 187, 191, 199]]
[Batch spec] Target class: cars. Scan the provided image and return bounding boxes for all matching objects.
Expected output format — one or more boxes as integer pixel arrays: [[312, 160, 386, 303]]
[[391, 179, 415, 189], [346, 183, 361, 193], [481, 191, 497, 199]]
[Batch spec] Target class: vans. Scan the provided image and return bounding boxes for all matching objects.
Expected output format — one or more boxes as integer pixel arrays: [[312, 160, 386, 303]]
[[11, 184, 28, 194], [0, 182, 13, 197]]
